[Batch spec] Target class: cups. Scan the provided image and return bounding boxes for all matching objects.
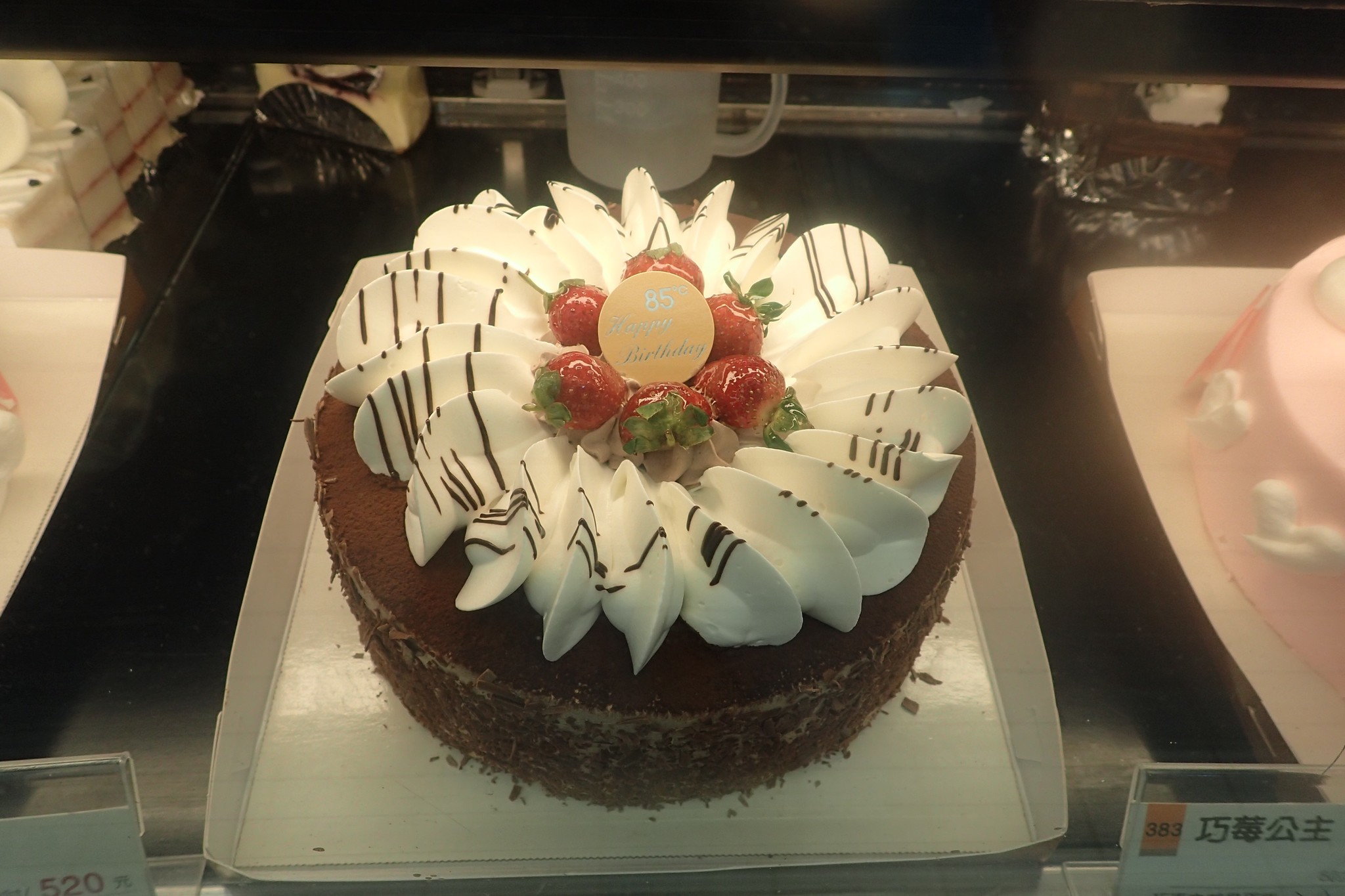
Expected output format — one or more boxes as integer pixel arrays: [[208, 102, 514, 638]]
[[559, 67, 789, 191]]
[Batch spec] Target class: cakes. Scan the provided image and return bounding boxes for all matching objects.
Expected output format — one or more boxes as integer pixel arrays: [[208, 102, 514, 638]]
[[1098, 84, 1243, 173], [304, 168, 978, 812], [1180, 233, 1345, 708], [0, 56, 188, 258]]
